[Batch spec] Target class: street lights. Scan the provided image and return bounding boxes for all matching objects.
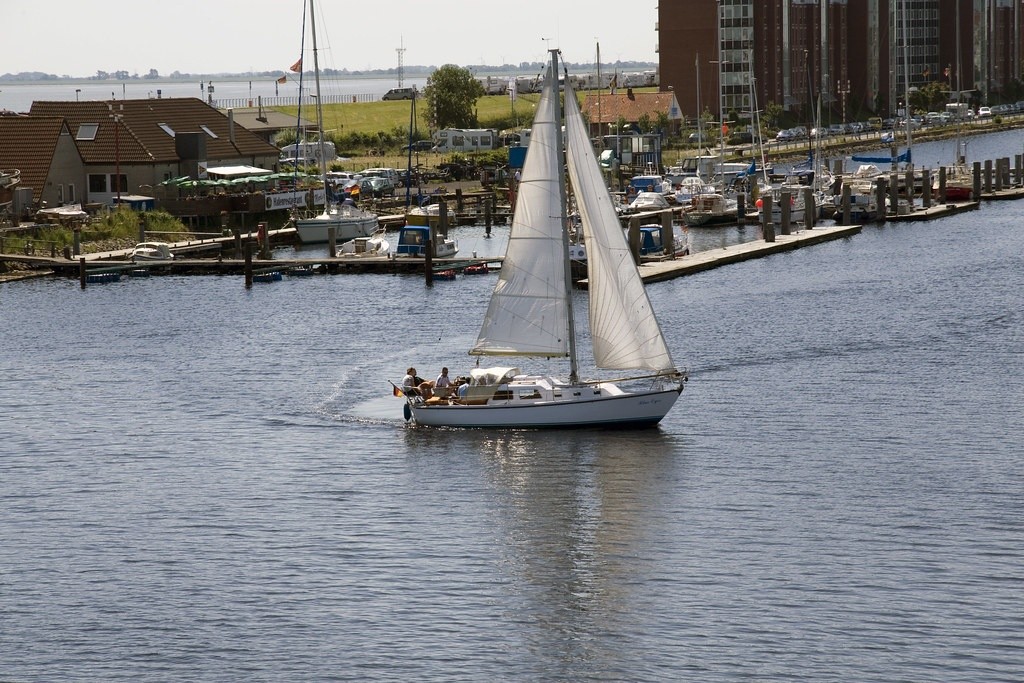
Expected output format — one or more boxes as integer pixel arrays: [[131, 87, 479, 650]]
[[506, 88, 515, 146], [668, 86, 676, 134], [311, 93, 326, 207]]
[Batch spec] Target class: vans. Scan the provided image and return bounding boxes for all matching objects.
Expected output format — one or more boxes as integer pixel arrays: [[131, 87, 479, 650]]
[[381, 88, 414, 101]]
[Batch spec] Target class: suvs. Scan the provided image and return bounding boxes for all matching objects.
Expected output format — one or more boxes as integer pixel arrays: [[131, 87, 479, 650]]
[[731, 132, 760, 143]]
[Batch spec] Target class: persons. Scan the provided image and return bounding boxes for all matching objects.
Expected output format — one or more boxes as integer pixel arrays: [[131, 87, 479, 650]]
[[435, 367, 453, 399], [402, 367, 434, 398], [515, 168, 521, 188], [457, 378, 471, 400]]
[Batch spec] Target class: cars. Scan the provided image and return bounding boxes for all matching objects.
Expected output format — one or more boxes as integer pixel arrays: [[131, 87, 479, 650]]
[[897, 118, 921, 131], [966, 109, 976, 119], [402, 141, 436, 153], [776, 130, 797, 142], [688, 133, 706, 144], [990, 100, 1024, 117], [978, 107, 992, 117], [810, 127, 828, 140], [323, 168, 416, 203], [828, 123, 845, 136], [940, 112, 955, 123]]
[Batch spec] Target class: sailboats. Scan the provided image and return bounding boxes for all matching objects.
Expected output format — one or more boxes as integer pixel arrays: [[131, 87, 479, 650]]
[[289, 0, 381, 244], [568, 152, 588, 283], [400, 38, 690, 427]]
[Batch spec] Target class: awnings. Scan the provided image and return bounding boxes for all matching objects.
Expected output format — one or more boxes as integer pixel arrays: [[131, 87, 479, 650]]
[[206, 165, 272, 178]]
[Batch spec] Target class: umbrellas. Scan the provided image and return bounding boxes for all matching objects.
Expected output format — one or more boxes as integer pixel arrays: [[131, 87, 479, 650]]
[[157, 172, 310, 193]]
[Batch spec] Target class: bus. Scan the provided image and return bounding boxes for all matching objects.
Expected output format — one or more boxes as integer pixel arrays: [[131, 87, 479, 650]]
[[945, 103, 969, 120]]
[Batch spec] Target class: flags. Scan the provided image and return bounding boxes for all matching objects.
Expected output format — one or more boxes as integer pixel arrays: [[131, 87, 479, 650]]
[[279, 75, 287, 84], [608, 76, 617, 90], [290, 58, 302, 72], [944, 65, 951, 76], [881, 130, 894, 143], [627, 89, 636, 102], [392, 385, 405, 398], [923, 67, 929, 77]]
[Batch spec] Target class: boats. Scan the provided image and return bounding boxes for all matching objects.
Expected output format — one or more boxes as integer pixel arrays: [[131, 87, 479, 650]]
[[128, 241, 174, 261], [253, 271, 282, 283], [405, 203, 458, 229], [638, 223, 691, 261], [336, 235, 389, 259], [393, 225, 459, 258], [609, 0, 983, 228]]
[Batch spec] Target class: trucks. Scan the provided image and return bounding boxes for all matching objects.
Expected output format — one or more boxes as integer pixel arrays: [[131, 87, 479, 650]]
[[431, 127, 499, 155]]
